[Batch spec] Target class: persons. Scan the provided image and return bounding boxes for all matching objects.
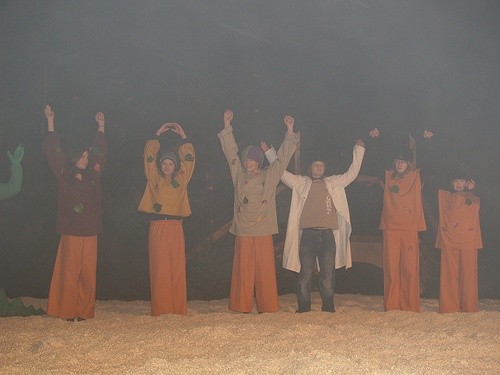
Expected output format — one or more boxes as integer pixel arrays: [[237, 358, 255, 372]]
[[366, 128, 434, 313], [434, 170, 483, 314], [259, 138, 365, 313], [44, 104, 108, 322], [0, 144, 24, 200], [218, 110, 300, 312], [137, 122, 195, 317]]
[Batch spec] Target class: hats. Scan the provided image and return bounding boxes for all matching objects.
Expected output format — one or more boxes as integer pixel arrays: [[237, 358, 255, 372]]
[[68, 145, 85, 165], [160, 152, 177, 167], [241, 145, 264, 163]]
